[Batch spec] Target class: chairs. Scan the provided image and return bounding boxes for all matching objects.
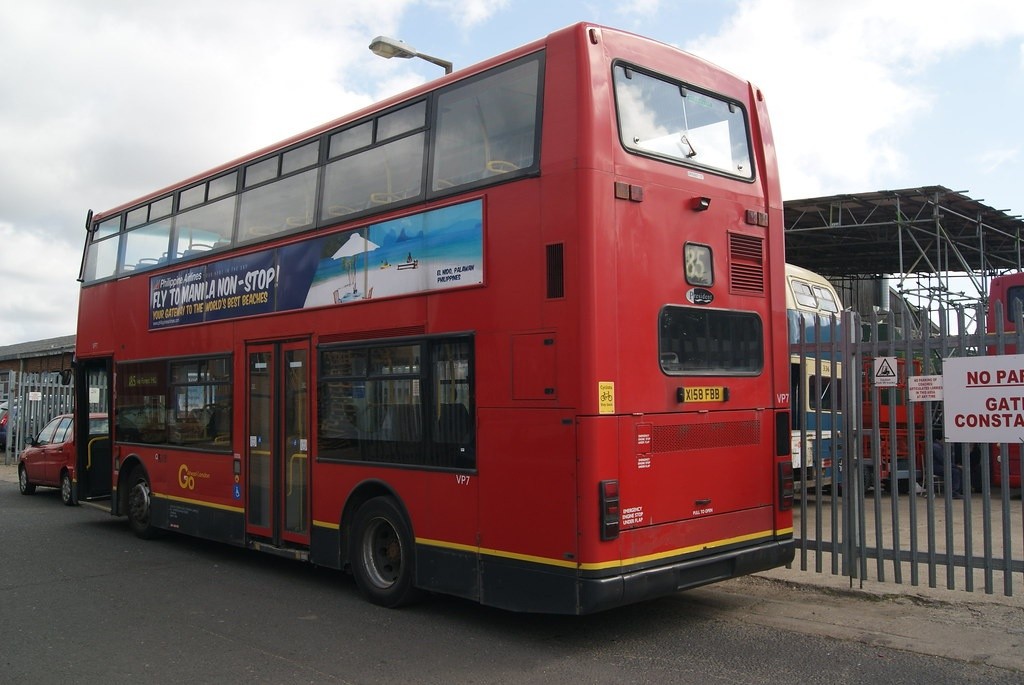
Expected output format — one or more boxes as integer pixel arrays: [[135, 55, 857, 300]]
[[114, 400, 472, 450], [111, 159, 522, 277]]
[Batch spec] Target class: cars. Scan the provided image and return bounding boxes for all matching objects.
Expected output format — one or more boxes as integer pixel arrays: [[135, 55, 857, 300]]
[[17, 413, 138, 507], [0, 395, 102, 453]]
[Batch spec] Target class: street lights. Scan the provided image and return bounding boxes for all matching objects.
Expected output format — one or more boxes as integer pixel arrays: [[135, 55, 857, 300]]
[[368, 33, 454, 78]]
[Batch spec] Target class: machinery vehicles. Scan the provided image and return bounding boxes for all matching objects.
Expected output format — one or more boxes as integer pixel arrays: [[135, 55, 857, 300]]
[[851, 352, 934, 495]]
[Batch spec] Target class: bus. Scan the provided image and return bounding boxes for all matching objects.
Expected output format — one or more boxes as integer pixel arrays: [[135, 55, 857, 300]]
[[69, 22, 793, 625], [783, 263, 857, 496], [987, 270, 1024, 492]]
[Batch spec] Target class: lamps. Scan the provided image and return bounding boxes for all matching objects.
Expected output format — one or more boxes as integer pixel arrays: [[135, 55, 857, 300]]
[[368, 35, 417, 60]]
